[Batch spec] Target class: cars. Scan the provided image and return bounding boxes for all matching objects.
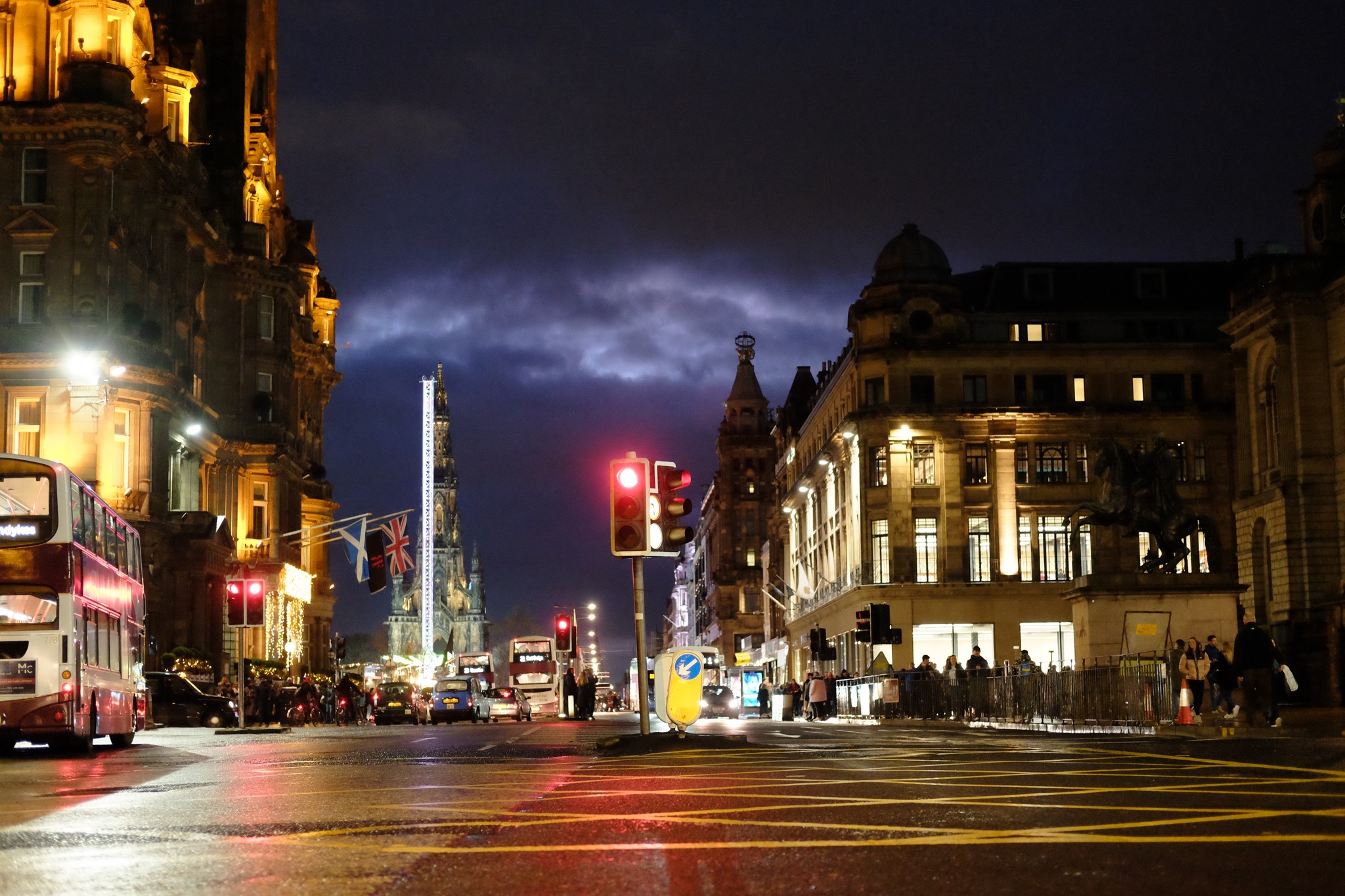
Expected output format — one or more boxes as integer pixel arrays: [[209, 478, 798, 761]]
[[702, 683, 739, 720], [431, 678, 490, 724], [486, 686, 533, 722]]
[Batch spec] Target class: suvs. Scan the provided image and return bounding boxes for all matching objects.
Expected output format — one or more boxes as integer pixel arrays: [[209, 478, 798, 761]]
[[144, 671, 239, 730], [372, 682, 427, 726]]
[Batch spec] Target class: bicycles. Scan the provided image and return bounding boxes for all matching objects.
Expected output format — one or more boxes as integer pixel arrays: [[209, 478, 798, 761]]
[[286, 699, 325, 728], [334, 693, 363, 725]]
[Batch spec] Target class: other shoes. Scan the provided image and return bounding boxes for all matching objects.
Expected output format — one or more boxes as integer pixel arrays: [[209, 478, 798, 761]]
[[1247, 716, 1255, 728], [1191, 706, 1203, 717], [574, 714, 595, 721], [1175, 716, 1178, 721], [1212, 706, 1224, 714], [1270, 717, 1282, 727], [1224, 705, 1240, 719], [227, 720, 270, 727], [307, 719, 342, 725], [1190, 714, 1203, 724], [1263, 713, 1270, 723], [759, 711, 837, 722]]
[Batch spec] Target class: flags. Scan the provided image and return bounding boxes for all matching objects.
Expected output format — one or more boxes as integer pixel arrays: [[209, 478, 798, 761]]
[[339, 517, 370, 584], [366, 529, 387, 595], [379, 513, 417, 578]]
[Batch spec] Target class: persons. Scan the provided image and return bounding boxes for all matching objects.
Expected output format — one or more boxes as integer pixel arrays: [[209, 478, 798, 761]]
[[1217, 642, 1240, 719], [216, 671, 432, 729], [1230, 611, 1285, 728], [1169, 639, 1195, 722], [1202, 635, 1226, 714], [756, 644, 1080, 724], [555, 666, 629, 721], [1179, 637, 1211, 723]]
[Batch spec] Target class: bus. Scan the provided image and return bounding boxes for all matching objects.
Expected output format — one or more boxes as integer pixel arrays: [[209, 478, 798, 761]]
[[509, 635, 560, 718], [1, 452, 142, 752], [444, 651, 496, 706]]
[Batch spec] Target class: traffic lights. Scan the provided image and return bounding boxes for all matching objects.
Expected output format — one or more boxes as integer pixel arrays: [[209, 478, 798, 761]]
[[649, 461, 694, 558], [874, 604, 893, 646], [854, 603, 875, 645], [609, 456, 651, 558], [553, 613, 572, 653], [245, 579, 265, 628], [226, 578, 247, 629], [810, 627, 832, 663]]
